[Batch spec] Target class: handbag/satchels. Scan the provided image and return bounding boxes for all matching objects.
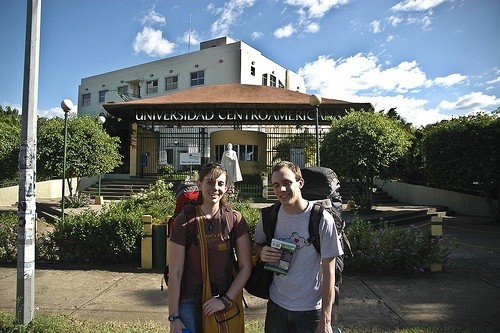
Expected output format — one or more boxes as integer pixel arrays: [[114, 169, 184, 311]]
[[243, 206, 272, 299], [203, 290, 248, 333]]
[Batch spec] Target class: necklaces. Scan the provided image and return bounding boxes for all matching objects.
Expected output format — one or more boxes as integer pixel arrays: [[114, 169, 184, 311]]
[[199, 206, 220, 231]]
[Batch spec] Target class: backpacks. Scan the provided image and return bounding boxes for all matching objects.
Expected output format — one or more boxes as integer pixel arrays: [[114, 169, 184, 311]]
[[272, 199, 344, 304], [164, 186, 236, 288]]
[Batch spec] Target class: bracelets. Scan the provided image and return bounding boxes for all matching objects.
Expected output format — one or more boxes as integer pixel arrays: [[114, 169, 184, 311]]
[[221, 295, 233, 308]]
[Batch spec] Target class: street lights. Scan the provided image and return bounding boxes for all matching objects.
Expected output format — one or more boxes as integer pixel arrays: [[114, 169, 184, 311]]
[[61, 99, 73, 223], [96, 115, 106, 205], [310, 93, 322, 166]]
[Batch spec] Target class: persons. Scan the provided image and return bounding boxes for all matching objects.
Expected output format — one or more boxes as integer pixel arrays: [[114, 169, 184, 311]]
[[220, 142, 243, 187], [254, 161, 344, 333], [167, 163, 254, 333]]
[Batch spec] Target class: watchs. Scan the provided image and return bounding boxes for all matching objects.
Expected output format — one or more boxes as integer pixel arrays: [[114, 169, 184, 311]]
[[168, 316, 179, 322]]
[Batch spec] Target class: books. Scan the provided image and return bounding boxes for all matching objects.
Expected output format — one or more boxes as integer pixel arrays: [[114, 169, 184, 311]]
[[263, 239, 296, 276]]
